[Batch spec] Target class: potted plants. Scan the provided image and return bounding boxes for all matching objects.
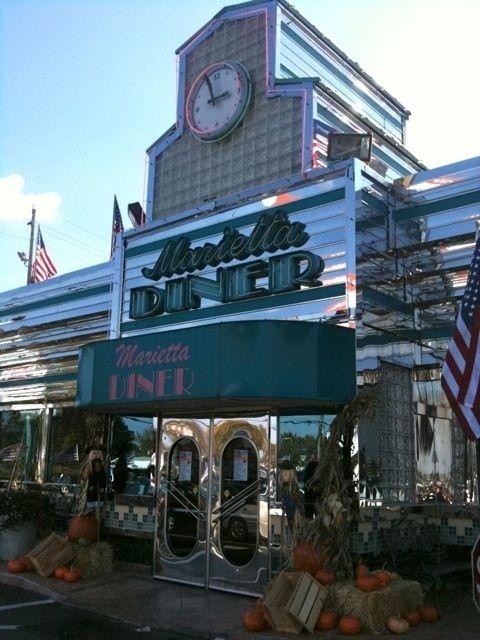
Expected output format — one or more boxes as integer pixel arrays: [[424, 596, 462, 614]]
[[0, 484, 55, 564], [262, 371, 388, 578], [64, 422, 109, 548]]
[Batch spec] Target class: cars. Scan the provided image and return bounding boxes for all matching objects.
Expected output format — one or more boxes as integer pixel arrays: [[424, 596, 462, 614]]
[[56, 465, 153, 497], [222, 487, 316, 542], [127, 478, 207, 535]]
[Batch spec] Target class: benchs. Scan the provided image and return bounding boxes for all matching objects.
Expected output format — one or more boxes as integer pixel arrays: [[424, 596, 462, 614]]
[[378, 521, 473, 610]]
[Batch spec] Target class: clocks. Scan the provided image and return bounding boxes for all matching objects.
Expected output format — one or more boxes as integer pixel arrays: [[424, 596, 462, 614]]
[[183, 59, 254, 145]]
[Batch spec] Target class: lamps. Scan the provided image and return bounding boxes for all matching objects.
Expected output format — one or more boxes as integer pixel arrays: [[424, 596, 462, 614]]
[[126, 201, 146, 228], [324, 130, 373, 164]]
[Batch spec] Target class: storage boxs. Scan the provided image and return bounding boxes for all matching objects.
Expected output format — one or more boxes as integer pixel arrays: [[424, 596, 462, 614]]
[[26, 531, 75, 578], [265, 569, 329, 635]]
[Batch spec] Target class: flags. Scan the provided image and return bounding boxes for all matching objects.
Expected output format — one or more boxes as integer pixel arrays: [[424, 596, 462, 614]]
[[442, 229, 480, 446], [33, 227, 57, 284], [110, 197, 123, 262]]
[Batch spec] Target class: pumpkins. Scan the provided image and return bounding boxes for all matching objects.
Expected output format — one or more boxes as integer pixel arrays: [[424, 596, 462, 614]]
[[386, 605, 438, 633], [356, 561, 392, 591], [243, 608, 266, 632], [315, 568, 335, 586], [55, 565, 84, 582], [8, 560, 24, 572], [316, 609, 337, 629], [291, 543, 323, 572], [68, 514, 97, 543], [339, 617, 361, 635]]
